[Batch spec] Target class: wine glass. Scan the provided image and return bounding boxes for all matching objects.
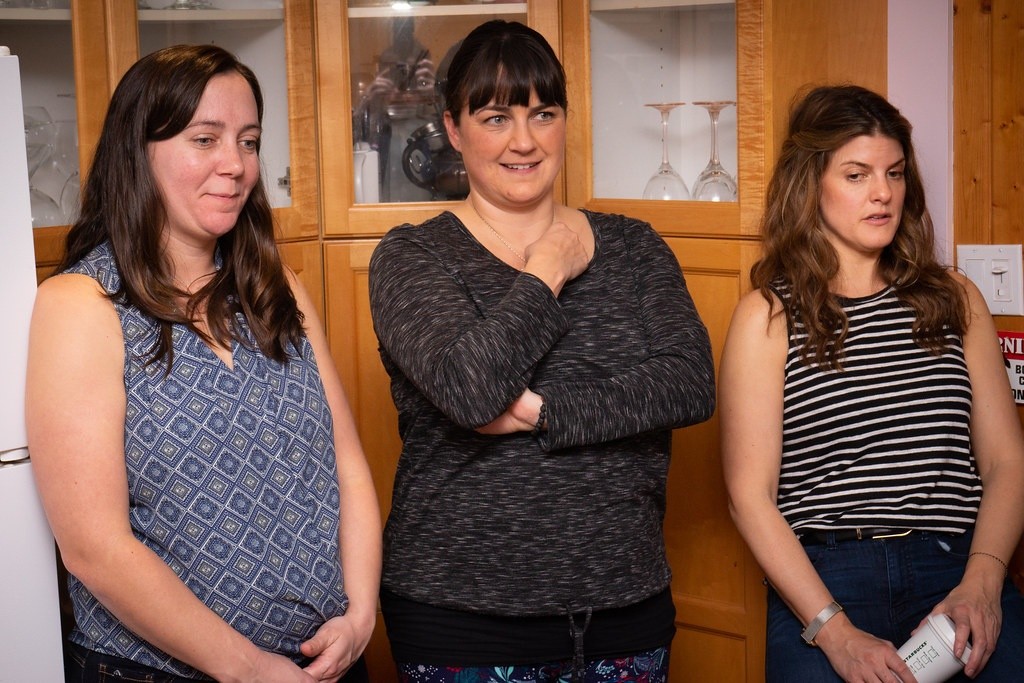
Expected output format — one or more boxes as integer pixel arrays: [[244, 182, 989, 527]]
[[638, 100, 690, 201], [691, 97, 737, 203]]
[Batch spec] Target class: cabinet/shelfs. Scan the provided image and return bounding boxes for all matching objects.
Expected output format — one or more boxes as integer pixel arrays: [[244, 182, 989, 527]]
[[0, 1, 1023, 683]]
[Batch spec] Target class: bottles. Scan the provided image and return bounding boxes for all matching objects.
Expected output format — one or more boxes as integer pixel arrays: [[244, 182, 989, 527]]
[[22, 105, 84, 226]]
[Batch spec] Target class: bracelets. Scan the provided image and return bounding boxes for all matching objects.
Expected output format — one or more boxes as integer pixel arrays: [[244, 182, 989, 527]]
[[801, 601, 843, 647], [530, 399, 546, 435], [967, 552, 1008, 577]]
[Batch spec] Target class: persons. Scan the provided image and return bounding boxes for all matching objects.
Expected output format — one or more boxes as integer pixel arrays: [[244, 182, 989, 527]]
[[25, 45, 383, 683], [366, 18, 717, 683], [713, 86, 1024, 683]]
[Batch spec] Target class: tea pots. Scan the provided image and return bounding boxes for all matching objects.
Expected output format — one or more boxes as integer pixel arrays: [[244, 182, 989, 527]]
[[402, 139, 472, 198]]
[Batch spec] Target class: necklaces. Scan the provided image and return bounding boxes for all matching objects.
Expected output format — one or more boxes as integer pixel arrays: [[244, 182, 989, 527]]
[[471, 201, 528, 264]]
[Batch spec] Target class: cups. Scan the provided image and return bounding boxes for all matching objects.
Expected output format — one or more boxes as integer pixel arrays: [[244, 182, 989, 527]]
[[888, 613, 972, 682], [353, 142, 380, 205]]
